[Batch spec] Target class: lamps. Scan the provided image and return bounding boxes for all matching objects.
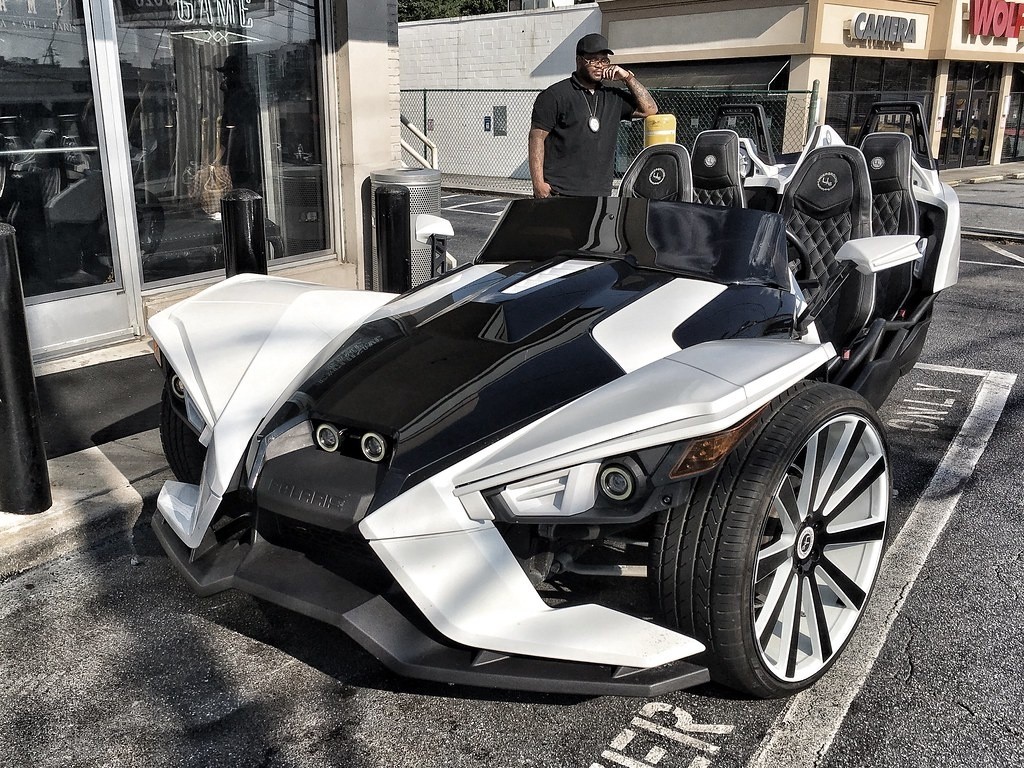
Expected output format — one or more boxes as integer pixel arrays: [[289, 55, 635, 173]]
[[181, 39, 233, 221]]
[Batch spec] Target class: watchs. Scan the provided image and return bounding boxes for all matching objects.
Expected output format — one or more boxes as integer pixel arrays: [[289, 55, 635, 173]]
[[622, 70, 635, 85]]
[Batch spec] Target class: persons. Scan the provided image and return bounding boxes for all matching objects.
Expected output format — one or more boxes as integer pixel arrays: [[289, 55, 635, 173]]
[[213, 56, 262, 193], [528, 33, 659, 197]]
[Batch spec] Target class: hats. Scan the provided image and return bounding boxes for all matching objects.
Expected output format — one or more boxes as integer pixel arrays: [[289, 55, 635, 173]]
[[576, 33, 614, 55]]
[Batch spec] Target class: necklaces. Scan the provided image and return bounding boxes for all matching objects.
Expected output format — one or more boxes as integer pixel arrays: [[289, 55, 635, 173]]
[[581, 88, 600, 133]]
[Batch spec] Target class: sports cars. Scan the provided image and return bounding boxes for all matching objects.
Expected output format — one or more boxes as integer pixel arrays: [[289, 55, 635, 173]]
[[0, 128, 163, 286], [146, 101, 958, 700]]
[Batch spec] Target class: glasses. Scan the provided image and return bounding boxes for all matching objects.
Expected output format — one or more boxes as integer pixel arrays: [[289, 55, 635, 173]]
[[581, 55, 610, 67]]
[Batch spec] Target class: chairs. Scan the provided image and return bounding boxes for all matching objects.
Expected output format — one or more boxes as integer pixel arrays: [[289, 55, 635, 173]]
[[778, 146, 877, 357], [859, 131, 919, 237], [690, 128, 747, 209], [617, 142, 694, 202]]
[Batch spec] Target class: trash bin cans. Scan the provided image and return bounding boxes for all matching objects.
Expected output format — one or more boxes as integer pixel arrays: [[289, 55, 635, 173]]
[[370, 168, 441, 294], [283, 165, 326, 256]]
